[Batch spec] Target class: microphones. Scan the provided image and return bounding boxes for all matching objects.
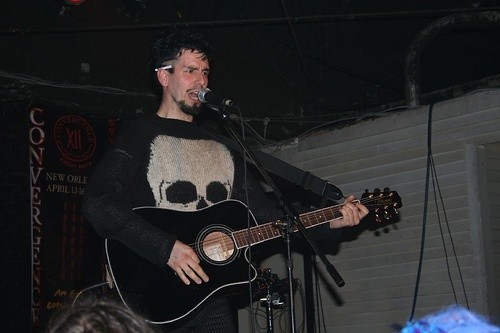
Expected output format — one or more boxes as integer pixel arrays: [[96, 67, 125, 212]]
[[198, 88, 241, 112]]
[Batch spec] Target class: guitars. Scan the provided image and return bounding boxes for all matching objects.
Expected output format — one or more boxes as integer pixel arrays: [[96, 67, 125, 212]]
[[105, 185, 404, 327]]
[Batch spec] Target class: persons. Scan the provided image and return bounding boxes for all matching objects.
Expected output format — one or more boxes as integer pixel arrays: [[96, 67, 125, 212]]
[[78, 33, 371, 332]]
